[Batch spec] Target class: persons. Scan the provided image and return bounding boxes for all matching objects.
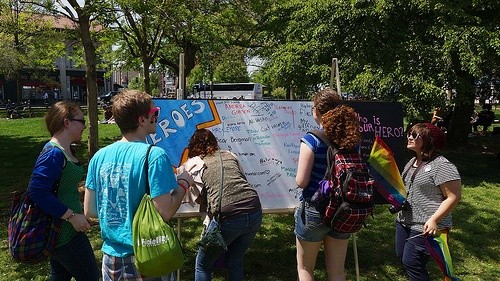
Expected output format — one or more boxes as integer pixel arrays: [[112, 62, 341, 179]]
[[187, 129, 262, 281], [472, 104, 495, 131], [84, 90, 194, 281], [431, 106, 454, 131], [293, 89, 360, 281], [394, 123, 461, 281], [104, 107, 114, 121], [28, 101, 101, 281], [5, 100, 23, 120]]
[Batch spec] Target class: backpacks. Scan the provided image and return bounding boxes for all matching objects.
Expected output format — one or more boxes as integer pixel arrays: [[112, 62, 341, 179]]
[[131, 145, 188, 278], [306, 129, 376, 233]]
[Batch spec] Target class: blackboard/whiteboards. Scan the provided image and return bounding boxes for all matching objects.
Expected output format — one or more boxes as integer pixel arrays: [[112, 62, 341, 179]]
[[137, 98, 405, 220]]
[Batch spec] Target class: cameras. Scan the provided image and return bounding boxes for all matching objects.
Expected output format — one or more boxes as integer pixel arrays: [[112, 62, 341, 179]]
[[389, 200, 411, 214]]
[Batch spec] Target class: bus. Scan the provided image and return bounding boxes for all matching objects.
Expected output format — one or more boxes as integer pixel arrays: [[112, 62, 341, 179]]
[[191, 82, 263, 99]]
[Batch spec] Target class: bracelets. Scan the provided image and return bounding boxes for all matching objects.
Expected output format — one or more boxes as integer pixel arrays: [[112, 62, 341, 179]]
[[178, 178, 190, 189], [66, 211, 75, 221], [177, 183, 187, 194]]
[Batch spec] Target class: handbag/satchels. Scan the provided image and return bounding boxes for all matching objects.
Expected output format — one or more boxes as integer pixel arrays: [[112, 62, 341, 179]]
[[423, 231, 462, 281], [196, 221, 228, 254], [7, 191, 60, 265], [366, 134, 407, 207]]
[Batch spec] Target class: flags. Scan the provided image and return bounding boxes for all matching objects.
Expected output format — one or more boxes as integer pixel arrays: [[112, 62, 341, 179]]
[[366, 135, 407, 210], [424, 227, 461, 281]]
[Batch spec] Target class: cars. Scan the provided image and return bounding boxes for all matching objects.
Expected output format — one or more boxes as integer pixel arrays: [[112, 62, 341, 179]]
[[100, 91, 122, 104]]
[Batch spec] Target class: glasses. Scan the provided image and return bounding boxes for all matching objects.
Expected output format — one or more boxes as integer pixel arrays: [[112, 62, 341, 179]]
[[144, 109, 158, 118], [67, 118, 85, 125], [408, 132, 424, 139]]
